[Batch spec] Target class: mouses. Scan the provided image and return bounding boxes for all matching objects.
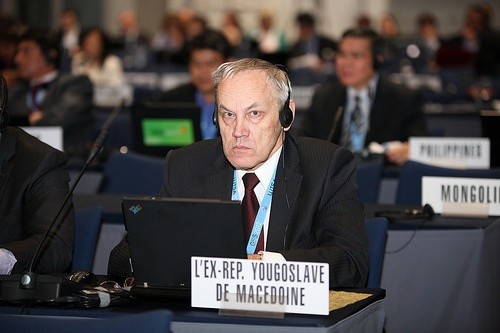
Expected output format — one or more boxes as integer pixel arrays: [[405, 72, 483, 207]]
[[67, 271, 98, 285]]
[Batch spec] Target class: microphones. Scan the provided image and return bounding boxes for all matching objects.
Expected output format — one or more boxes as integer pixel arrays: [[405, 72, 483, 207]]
[[327, 105, 344, 141], [21, 129, 107, 290]]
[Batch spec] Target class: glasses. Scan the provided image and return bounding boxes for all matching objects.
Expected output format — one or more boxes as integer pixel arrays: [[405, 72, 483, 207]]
[[99, 277, 135, 290]]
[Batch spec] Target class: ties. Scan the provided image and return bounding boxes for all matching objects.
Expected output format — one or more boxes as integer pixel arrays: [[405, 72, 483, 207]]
[[241, 173, 264, 254], [30, 81, 50, 110], [346, 97, 361, 153]]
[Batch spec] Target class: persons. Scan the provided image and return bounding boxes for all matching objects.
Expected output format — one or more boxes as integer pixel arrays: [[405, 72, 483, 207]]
[[0, 74, 75, 277], [108, 58, 372, 291], [0, 0, 500, 165]]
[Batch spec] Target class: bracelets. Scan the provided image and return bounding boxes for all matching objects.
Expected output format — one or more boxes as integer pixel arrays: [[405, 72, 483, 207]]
[[259, 253, 263, 260]]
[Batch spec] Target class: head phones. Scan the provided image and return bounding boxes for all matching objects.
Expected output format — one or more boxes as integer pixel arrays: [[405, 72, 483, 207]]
[[374, 203, 434, 221], [213, 73, 293, 127]]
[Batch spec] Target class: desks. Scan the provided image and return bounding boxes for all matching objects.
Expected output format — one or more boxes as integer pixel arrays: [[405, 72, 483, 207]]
[[0, 104, 500, 333]]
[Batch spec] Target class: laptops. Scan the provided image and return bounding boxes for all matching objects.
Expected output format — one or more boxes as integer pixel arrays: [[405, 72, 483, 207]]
[[121, 196, 247, 299]]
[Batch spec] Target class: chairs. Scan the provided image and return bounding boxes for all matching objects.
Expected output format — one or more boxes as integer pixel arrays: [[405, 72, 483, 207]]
[[94, 148, 166, 194], [97, 116, 132, 148], [358, 157, 385, 204], [363, 216, 388, 288], [394, 160, 500, 208], [68, 204, 104, 273]]
